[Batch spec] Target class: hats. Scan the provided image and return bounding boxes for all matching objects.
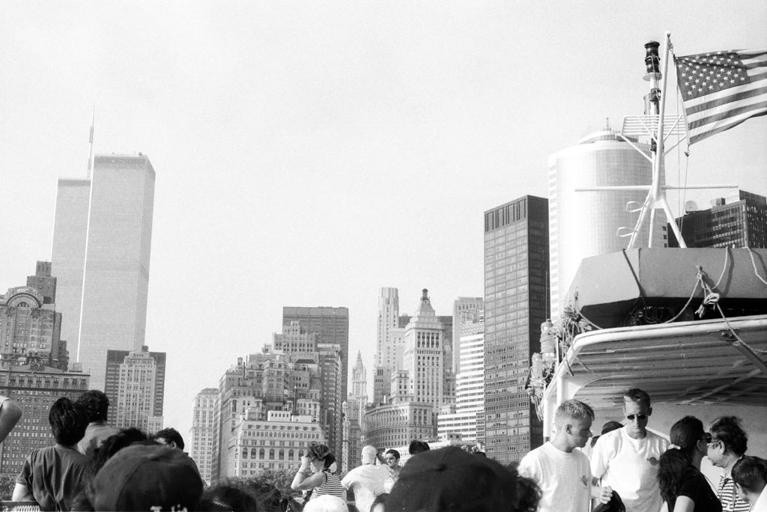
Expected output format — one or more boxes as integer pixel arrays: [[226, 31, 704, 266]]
[[362, 445, 376, 464], [94, 445, 203, 511]]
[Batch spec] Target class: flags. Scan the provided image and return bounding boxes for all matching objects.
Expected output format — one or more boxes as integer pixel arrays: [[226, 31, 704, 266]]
[[675, 48, 766, 145]]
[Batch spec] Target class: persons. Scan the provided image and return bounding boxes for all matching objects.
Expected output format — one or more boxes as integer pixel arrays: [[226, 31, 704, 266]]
[[13, 390, 257, 512], [291, 440, 430, 512], [516, 388, 767, 512], [0, 394, 22, 443]]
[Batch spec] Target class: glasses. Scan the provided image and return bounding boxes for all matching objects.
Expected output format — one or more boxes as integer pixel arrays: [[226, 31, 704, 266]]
[[701, 433, 712, 444], [627, 413, 649, 420]]
